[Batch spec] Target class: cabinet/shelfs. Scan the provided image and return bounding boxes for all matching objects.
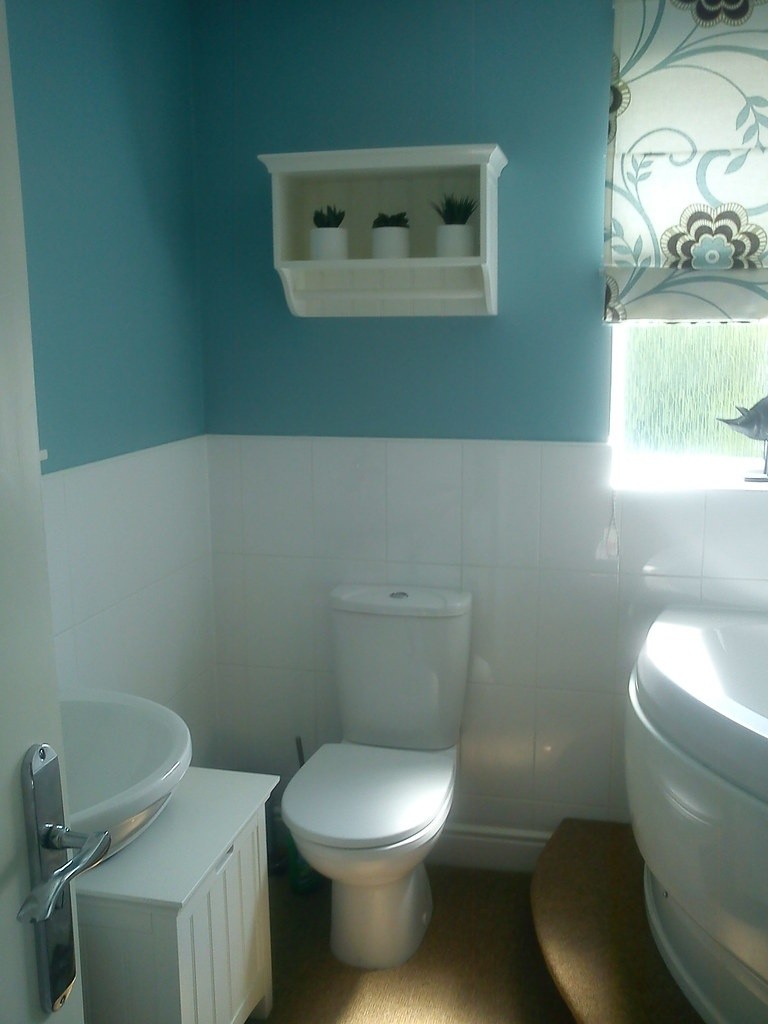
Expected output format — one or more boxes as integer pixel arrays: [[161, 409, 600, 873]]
[[74, 762, 282, 1024]]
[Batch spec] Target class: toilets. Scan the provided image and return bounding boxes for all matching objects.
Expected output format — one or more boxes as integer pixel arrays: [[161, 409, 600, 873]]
[[277, 578, 475, 972]]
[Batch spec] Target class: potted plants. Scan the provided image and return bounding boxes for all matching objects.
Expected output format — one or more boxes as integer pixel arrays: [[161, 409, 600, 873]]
[[307, 189, 478, 262]]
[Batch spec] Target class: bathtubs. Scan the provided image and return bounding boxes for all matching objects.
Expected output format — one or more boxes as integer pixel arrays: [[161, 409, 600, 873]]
[[622, 604, 768, 1024]]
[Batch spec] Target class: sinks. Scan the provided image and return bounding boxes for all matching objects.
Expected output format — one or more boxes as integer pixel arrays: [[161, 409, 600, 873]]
[[57, 688, 194, 865]]
